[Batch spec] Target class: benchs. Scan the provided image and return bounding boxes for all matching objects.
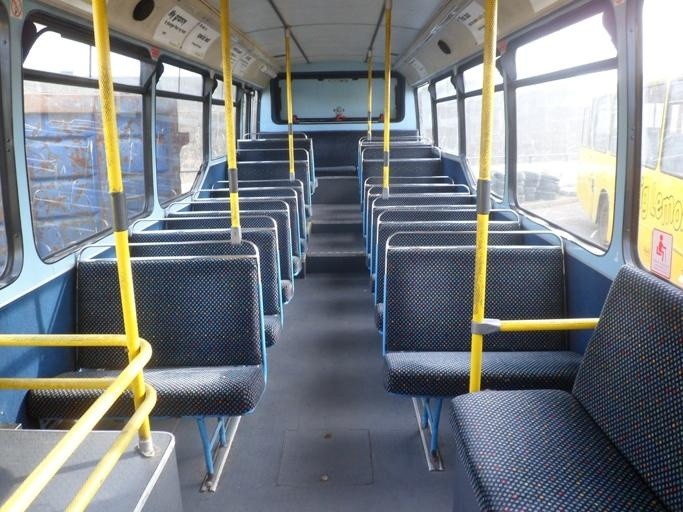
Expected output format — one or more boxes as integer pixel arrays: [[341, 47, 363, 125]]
[[13, 132, 316, 493], [357, 134, 682, 512]]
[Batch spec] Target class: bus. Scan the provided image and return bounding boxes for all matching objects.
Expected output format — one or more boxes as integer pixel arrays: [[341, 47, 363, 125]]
[[574, 77, 682, 288]]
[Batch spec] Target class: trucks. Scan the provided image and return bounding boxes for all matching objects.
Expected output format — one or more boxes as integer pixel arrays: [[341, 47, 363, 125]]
[[0, 88, 189, 281]]
